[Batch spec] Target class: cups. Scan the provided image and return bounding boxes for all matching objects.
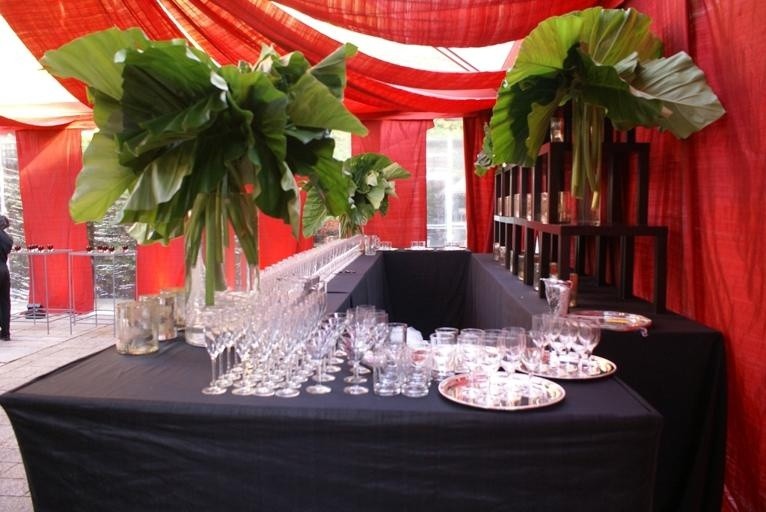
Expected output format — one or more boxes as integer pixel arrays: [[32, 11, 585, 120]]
[[118, 296, 159, 354], [364, 234, 379, 257], [418, 240, 425, 250], [153, 288, 184, 341], [381, 241, 393, 252], [412, 241, 417, 250], [374, 344, 431, 400]]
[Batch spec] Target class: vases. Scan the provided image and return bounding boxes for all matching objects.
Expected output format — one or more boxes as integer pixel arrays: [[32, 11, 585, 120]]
[[312, 217, 342, 248], [184, 146, 259, 349]]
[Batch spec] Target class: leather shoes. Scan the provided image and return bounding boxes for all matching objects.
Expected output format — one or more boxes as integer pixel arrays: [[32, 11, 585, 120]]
[[0, 331, 10, 341]]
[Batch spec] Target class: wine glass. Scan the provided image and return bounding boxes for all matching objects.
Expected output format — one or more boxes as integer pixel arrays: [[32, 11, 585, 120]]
[[458, 327, 484, 374], [307, 306, 384, 396], [531, 314, 555, 358], [499, 327, 526, 377], [570, 316, 601, 368], [260, 234, 360, 291], [195, 279, 325, 395], [545, 318, 574, 368], [430, 327, 458, 378], [482, 328, 502, 377], [545, 280, 572, 318], [387, 323, 408, 360]]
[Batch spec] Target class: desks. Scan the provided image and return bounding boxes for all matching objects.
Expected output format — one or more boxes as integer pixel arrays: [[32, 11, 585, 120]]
[[18, 252, 728, 512]]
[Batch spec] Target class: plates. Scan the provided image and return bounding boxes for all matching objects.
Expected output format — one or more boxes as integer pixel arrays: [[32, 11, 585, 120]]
[[437, 373, 565, 409], [566, 309, 651, 331], [518, 351, 616, 380]]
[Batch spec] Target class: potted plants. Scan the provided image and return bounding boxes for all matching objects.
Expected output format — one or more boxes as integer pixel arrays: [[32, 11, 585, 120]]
[[38, 30, 368, 346], [488, 9, 725, 226], [296, 153, 409, 237]]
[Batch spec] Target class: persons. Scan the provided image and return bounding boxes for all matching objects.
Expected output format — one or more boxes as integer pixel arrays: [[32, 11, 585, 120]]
[[0, 214, 13, 342]]
